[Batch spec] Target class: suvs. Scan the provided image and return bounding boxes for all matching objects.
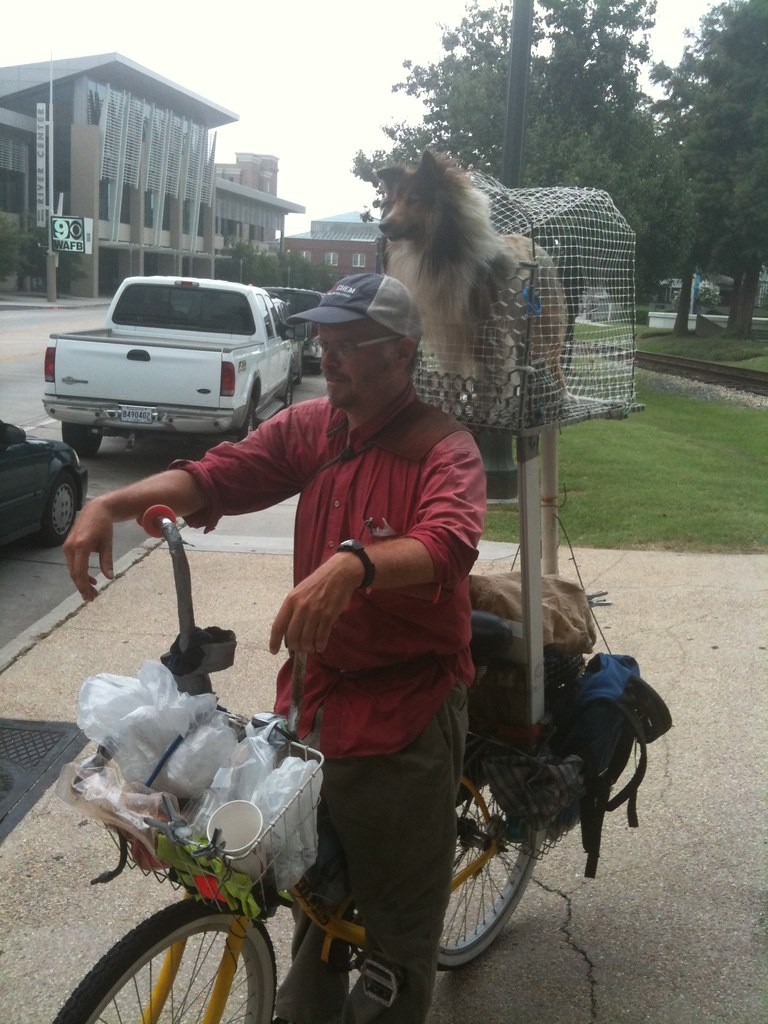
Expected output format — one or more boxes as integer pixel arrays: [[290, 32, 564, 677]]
[[255, 285, 330, 377]]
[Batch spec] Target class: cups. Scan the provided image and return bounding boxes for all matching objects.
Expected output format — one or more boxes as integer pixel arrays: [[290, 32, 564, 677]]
[[206, 800, 266, 882]]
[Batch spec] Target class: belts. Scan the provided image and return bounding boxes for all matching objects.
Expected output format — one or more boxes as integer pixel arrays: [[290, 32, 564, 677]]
[[319, 646, 436, 678]]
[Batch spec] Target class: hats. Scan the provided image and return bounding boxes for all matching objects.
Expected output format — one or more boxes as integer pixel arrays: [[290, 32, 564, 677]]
[[284, 273, 424, 350]]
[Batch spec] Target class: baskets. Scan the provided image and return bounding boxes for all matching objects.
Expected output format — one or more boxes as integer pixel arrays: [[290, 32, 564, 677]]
[[69, 707, 324, 919]]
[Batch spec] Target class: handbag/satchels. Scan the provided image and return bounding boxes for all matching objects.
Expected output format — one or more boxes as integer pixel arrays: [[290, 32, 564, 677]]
[[551, 651, 677, 822]]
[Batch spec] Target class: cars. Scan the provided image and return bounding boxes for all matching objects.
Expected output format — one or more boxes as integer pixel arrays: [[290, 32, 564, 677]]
[[0, 419, 89, 549]]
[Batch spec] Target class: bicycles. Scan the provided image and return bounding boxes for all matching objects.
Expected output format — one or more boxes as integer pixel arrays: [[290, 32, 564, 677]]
[[55, 505, 586, 1024]]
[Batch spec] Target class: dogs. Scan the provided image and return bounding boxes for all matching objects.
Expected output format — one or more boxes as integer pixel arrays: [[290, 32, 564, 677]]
[[373, 147, 568, 418]]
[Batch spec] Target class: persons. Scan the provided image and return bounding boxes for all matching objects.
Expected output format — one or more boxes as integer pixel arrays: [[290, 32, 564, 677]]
[[64, 273, 486, 1024]]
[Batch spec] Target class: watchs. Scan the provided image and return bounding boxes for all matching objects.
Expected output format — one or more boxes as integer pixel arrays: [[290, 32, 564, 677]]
[[337, 539, 374, 588]]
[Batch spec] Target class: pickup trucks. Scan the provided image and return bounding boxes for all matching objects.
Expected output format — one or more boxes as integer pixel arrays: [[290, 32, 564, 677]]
[[44, 275, 308, 459]]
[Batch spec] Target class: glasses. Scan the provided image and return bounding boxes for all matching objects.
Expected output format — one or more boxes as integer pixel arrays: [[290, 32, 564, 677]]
[[315, 331, 403, 358]]
